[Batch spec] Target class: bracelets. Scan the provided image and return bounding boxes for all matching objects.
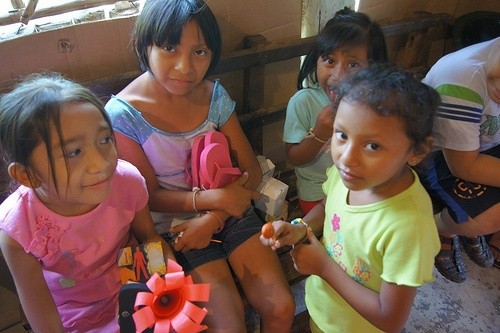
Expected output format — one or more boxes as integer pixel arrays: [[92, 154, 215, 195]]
[[191, 186, 200, 214], [304, 128, 330, 146], [203, 211, 224, 232], [290, 218, 311, 243]]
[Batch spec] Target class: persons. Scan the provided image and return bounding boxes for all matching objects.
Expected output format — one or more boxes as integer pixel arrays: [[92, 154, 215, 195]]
[[260, 64, 442, 333], [0, 0, 295, 333], [411, 38, 500, 283], [282, 6, 389, 215]]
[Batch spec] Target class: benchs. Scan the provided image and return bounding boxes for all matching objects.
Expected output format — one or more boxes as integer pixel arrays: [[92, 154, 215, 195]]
[[0, 14, 492, 333]]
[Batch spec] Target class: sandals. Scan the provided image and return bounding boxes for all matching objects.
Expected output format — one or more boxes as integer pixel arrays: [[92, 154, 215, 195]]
[[435, 236, 467, 283], [458, 236, 495, 267]]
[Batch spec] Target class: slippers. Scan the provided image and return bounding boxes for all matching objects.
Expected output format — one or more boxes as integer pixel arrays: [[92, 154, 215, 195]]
[[184, 132, 204, 192], [200, 143, 242, 189], [204, 130, 229, 155]]
